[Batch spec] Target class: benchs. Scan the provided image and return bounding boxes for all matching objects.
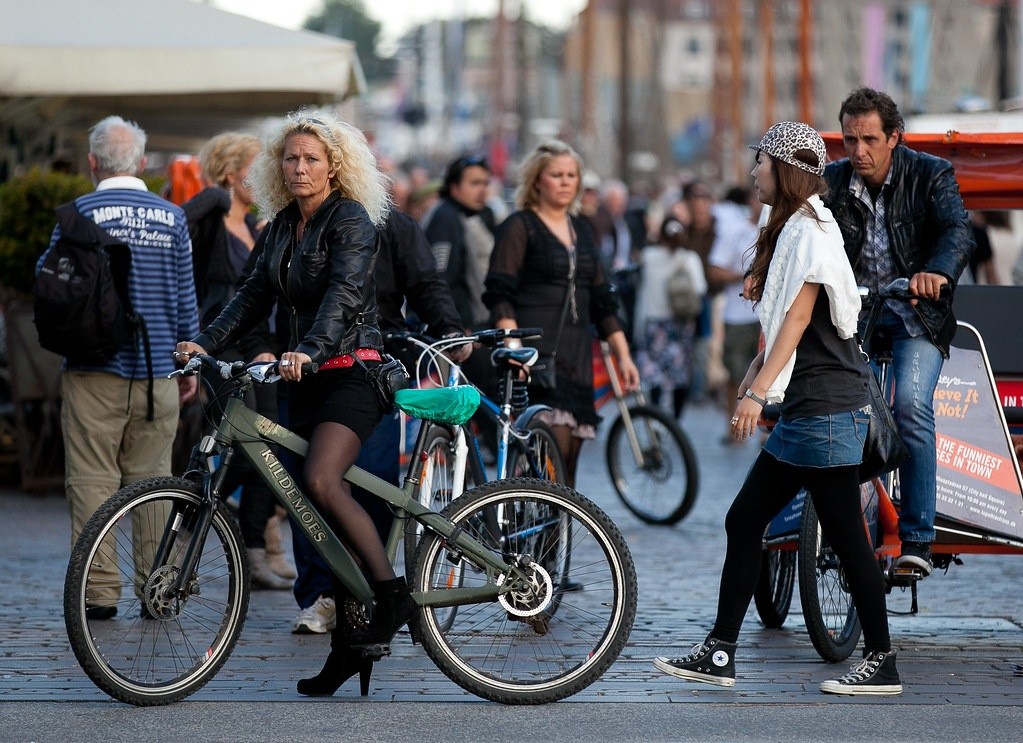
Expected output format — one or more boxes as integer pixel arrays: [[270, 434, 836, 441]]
[[944, 285, 1023, 416]]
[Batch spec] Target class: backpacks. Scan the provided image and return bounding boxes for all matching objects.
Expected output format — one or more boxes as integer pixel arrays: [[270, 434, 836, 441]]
[[31, 200, 138, 369]]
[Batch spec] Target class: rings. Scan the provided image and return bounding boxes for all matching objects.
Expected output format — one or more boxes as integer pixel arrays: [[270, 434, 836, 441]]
[[736, 397, 743, 400], [730, 417, 739, 426], [173, 351, 189, 358], [281, 359, 295, 366]]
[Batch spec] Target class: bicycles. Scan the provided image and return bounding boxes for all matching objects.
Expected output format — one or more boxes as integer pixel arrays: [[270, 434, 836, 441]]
[[380, 327, 570, 643], [64, 351, 638, 705]]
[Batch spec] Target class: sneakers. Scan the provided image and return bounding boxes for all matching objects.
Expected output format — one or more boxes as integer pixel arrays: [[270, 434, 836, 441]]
[[820, 648, 902, 696], [895, 540, 934, 578], [654, 636, 740, 687]]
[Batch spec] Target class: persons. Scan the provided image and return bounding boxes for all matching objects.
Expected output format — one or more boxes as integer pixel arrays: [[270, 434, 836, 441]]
[[176, 107, 422, 696], [653, 121, 903, 693], [35, 116, 201, 620], [481, 141, 641, 592], [743, 85, 977, 575], [184, 130, 1000, 631]]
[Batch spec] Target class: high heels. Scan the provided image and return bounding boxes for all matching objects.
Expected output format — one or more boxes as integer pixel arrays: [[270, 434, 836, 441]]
[[296, 628, 373, 699], [359, 579, 421, 659]]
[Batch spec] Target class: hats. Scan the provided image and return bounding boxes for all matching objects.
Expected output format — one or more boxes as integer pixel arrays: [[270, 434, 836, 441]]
[[748, 122, 827, 177]]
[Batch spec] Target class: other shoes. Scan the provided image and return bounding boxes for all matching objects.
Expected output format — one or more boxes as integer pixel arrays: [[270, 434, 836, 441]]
[[264, 517, 297, 578], [245, 548, 294, 592]]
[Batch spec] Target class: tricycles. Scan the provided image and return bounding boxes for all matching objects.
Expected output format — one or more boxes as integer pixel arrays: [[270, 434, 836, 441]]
[[592, 262, 700, 526], [738, 279, 1023, 663]]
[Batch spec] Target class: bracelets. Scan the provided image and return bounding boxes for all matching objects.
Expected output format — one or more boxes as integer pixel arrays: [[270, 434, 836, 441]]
[[746, 389, 768, 408]]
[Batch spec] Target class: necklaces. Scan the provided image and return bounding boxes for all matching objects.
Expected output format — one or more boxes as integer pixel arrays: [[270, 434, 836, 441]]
[[297, 218, 302, 246]]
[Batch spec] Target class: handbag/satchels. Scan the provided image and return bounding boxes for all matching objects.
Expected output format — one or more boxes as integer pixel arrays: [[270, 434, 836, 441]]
[[668, 266, 700, 320], [524, 351, 558, 393], [365, 352, 408, 414], [846, 356, 911, 487]]
